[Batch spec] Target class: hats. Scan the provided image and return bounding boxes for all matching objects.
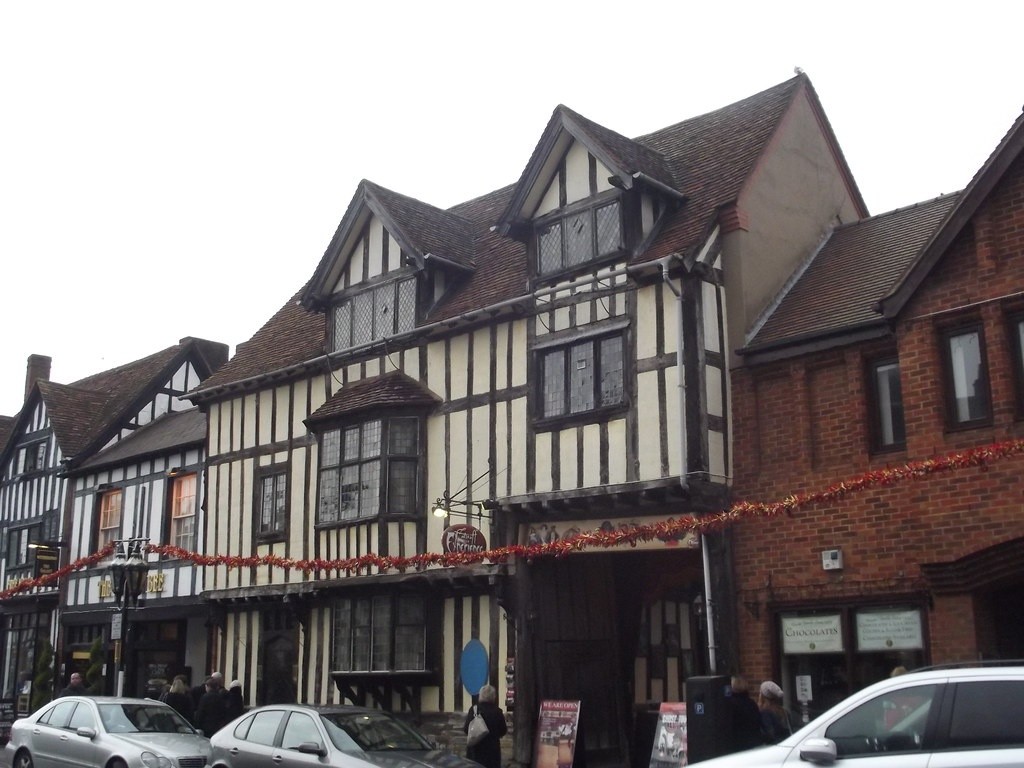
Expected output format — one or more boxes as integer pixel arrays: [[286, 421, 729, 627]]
[[760, 680, 784, 701]]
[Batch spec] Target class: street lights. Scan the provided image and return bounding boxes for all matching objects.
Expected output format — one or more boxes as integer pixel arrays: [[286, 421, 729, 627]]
[[109, 536, 151, 699]]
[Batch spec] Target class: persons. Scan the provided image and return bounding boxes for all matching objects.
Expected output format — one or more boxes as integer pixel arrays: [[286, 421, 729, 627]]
[[464, 686, 506, 767], [230, 679, 245, 720], [211, 672, 236, 725], [163, 675, 194, 727], [194, 678, 224, 739], [59, 673, 87, 698], [730, 676, 762, 754], [758, 682, 792, 747]]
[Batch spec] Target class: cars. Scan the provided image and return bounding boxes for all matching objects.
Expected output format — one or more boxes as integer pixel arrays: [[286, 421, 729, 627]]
[[2, 695, 211, 768], [681, 663, 1023, 768], [206, 701, 481, 768]]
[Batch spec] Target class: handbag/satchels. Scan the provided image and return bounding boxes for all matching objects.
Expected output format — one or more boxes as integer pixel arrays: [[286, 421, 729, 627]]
[[466, 705, 489, 748]]
